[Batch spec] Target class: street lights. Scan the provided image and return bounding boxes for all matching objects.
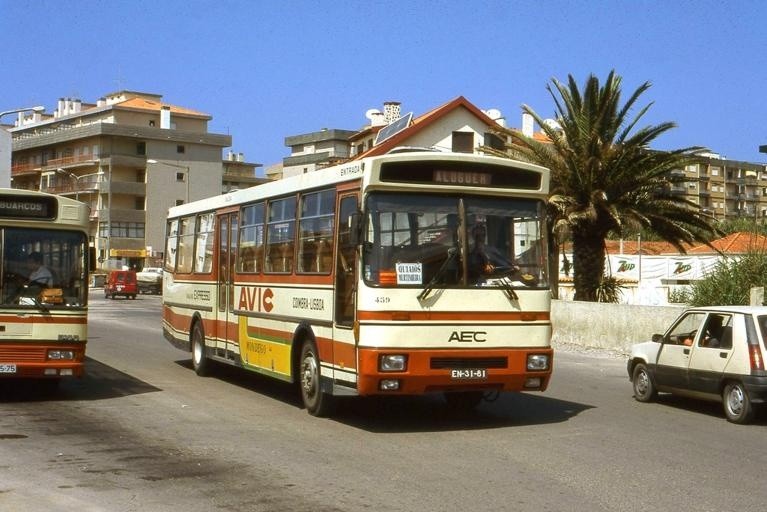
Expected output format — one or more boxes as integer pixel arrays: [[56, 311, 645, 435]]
[[57, 168, 105, 200], [0, 106, 46, 118]]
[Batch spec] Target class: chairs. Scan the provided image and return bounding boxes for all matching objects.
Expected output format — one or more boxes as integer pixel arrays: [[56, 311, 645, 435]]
[[220, 229, 355, 324], [46, 267, 60, 285]]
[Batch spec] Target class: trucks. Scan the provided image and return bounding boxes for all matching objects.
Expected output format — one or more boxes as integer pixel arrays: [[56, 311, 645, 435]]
[[136, 268, 163, 295]]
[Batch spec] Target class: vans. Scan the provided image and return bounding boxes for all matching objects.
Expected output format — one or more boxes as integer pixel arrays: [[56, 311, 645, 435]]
[[105, 268, 137, 299]]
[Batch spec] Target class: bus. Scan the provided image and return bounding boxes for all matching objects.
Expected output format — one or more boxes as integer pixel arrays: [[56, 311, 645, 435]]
[[1, 188, 96, 379], [163, 146, 553, 417]]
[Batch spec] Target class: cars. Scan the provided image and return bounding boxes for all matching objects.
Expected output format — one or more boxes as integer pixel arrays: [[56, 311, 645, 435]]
[[627, 306, 767, 423]]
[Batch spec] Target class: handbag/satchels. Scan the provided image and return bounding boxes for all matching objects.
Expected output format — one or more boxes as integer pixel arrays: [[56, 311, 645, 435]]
[[40, 288, 64, 304]]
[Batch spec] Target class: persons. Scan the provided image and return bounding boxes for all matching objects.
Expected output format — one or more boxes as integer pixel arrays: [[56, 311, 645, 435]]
[[26, 251, 53, 289], [466, 224, 518, 284], [683, 316, 723, 347]]
